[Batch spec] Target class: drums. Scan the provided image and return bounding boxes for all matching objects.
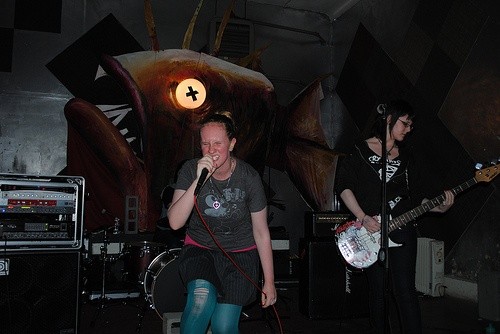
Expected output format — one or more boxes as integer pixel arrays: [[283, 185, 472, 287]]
[[123, 242, 164, 278], [144, 247, 188, 327]]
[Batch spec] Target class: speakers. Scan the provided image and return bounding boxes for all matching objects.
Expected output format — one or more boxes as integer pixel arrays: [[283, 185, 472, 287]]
[[297, 238, 371, 322], [0, 252, 80, 334]]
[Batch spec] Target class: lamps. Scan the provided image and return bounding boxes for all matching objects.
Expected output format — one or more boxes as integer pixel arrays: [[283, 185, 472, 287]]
[[176, 78, 207, 109]]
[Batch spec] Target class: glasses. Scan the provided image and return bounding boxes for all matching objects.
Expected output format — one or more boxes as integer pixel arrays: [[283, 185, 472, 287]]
[[398, 119, 415, 130]]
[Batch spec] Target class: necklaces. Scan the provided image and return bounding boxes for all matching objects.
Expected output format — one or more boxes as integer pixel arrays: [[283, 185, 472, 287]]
[[209, 167, 233, 209], [379, 140, 396, 158]]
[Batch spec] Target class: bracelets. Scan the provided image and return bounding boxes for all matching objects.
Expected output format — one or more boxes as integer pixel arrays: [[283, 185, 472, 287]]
[[361, 215, 366, 225]]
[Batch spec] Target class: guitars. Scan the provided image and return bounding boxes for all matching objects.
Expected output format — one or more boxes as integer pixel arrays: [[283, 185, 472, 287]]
[[333, 160, 500, 274]]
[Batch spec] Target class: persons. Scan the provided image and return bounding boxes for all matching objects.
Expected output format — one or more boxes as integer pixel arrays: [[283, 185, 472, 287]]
[[333, 102, 454, 334], [167, 112, 277, 334]]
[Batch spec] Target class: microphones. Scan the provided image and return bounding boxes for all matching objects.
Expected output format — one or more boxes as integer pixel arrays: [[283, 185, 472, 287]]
[[192, 168, 209, 195]]
